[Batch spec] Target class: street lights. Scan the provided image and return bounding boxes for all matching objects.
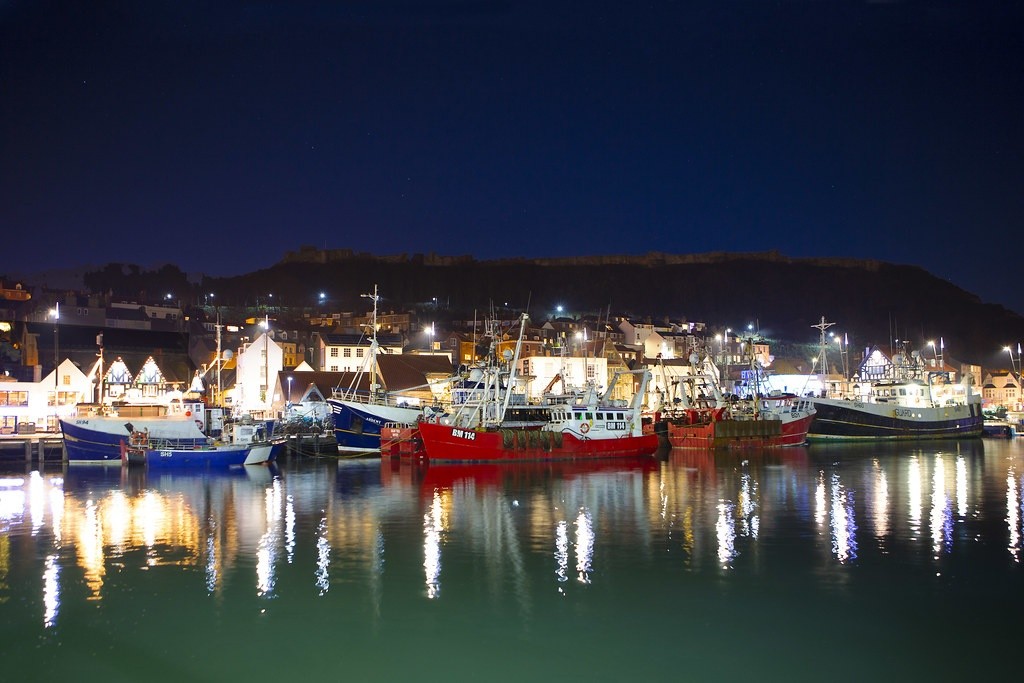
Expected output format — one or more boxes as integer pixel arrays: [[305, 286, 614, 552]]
[[287, 377, 292, 421]]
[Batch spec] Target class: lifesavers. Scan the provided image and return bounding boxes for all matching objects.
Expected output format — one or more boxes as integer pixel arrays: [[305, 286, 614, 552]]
[[580, 423, 590, 434], [131, 432, 146, 445]]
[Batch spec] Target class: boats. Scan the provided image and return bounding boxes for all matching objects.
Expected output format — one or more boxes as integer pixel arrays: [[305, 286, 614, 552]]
[[56, 280, 1015, 459], [123, 423, 274, 467]]
